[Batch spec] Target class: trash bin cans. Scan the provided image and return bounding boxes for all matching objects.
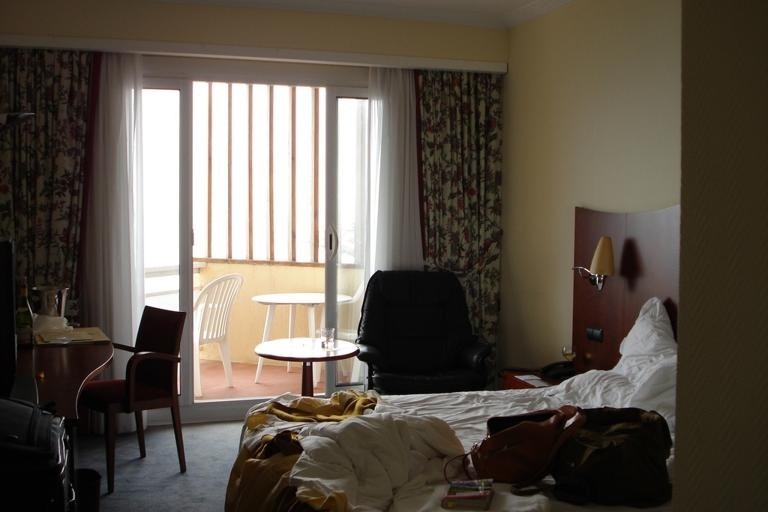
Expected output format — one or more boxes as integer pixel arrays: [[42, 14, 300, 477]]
[[540, 361, 577, 385], [69, 468, 102, 512]]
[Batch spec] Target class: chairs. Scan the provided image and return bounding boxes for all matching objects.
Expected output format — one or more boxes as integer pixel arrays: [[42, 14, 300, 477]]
[[354, 269, 487, 397], [80, 305, 186, 495], [318, 282, 364, 385], [192, 274, 244, 398]]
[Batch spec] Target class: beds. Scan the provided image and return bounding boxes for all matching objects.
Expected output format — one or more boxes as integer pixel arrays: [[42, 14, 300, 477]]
[[224, 206, 680, 512]]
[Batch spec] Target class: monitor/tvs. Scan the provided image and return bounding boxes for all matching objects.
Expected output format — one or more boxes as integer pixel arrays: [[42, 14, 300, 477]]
[[0, 239, 40, 407]]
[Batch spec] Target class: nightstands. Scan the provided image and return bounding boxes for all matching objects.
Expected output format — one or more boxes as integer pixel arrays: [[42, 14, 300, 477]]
[[499, 369, 551, 390]]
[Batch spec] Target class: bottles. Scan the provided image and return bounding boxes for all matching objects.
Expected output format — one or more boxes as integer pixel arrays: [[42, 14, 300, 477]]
[[17, 275, 33, 329]]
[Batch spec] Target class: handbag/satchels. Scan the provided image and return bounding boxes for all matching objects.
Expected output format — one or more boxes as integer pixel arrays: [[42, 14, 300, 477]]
[[469, 415, 560, 484], [510, 409, 671, 509]]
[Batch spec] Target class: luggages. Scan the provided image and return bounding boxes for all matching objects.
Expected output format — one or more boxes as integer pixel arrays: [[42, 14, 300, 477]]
[[1, 396, 78, 511]]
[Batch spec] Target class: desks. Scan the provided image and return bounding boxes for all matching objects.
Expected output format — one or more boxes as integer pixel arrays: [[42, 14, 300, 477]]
[[0, 326, 115, 478]]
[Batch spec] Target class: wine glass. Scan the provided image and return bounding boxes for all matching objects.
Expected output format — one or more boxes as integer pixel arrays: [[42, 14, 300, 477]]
[[561, 343, 577, 363]]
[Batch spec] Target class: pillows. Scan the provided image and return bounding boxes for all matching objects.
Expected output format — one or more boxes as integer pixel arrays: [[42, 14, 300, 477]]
[[619, 297, 677, 356]]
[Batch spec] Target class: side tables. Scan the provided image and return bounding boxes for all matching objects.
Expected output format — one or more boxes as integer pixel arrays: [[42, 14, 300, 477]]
[[252, 293, 353, 385], [253, 337, 361, 398]]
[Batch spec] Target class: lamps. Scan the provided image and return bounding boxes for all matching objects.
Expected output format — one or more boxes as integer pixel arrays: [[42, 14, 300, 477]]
[[572, 235, 615, 293]]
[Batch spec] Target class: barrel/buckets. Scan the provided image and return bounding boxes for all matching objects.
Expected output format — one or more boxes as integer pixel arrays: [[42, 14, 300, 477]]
[[25, 285, 70, 318]]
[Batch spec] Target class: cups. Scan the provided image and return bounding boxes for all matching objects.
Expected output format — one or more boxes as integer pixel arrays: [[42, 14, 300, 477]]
[[321, 327, 336, 349]]
